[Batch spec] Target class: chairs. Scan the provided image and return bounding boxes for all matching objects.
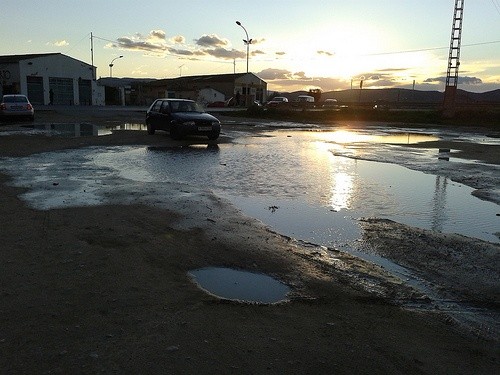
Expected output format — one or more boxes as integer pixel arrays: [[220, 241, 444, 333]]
[[177, 103, 184, 110]]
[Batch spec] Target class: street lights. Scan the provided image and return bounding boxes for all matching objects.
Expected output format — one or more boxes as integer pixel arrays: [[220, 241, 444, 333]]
[[109, 56, 124, 77], [236, 21, 252, 73]]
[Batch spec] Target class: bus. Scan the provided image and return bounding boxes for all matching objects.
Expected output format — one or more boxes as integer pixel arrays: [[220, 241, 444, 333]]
[[298, 96, 315, 103]]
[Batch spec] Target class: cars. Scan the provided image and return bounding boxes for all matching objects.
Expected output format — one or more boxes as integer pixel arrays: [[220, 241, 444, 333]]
[[267, 97, 289, 106], [207, 100, 228, 108], [146, 98, 221, 142], [322, 99, 339, 110], [251, 99, 265, 111], [0, 95, 35, 121]]
